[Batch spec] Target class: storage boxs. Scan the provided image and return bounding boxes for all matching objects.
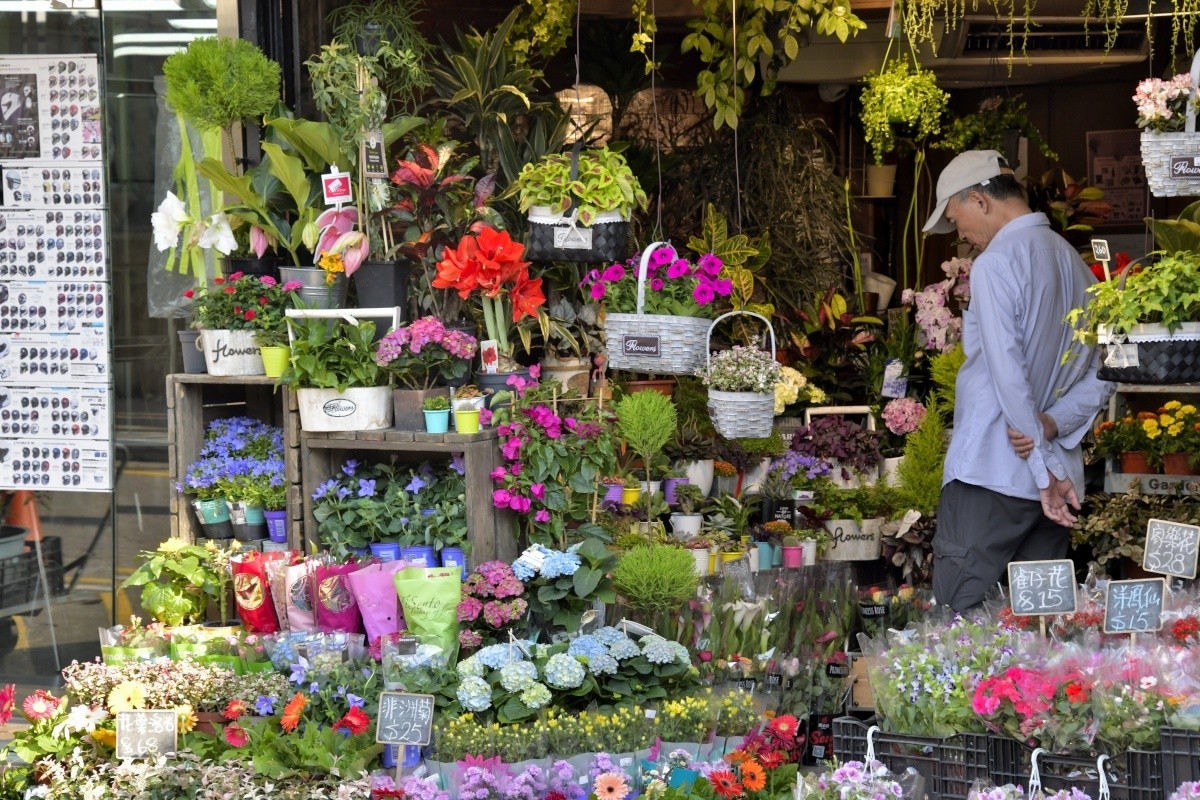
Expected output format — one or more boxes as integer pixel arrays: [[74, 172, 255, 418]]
[[162, 372, 305, 554], [0, 535, 65, 608], [297, 428, 519, 564], [830, 713, 1200, 800]]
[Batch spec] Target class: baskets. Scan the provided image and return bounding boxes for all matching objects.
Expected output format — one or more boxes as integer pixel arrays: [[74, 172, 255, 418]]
[[706, 311, 776, 441], [528, 142, 631, 262], [1096, 257, 1200, 384], [604, 242, 712, 375], [1140, 48, 1200, 198]]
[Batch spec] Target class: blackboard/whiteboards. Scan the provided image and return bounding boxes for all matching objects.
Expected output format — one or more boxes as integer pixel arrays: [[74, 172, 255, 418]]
[[1143, 519, 1199, 580], [116, 710, 177, 760], [376, 691, 434, 746], [1104, 577, 1165, 634], [1008, 559, 1077, 617]]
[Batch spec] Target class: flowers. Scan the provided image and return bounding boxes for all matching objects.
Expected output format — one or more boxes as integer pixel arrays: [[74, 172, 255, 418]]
[[0, 541, 797, 799], [802, 754, 904, 800], [860, 582, 1200, 747], [1168, 780, 1200, 800], [148, 38, 1200, 526], [970, 783, 1092, 800]]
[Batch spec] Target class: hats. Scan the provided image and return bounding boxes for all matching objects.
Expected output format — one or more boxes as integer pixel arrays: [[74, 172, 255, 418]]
[[921, 150, 1014, 234]]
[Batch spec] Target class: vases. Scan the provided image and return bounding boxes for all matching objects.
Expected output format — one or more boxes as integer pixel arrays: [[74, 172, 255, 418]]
[[176, 159, 1200, 800]]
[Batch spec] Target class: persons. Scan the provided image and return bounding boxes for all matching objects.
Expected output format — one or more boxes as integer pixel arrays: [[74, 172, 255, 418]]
[[921, 150, 1122, 621]]
